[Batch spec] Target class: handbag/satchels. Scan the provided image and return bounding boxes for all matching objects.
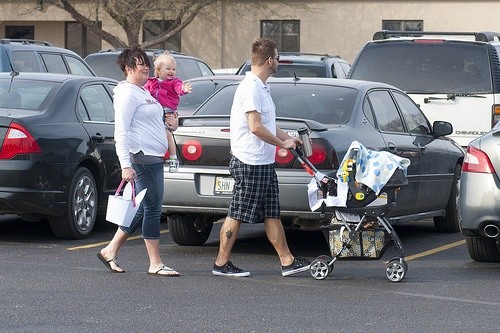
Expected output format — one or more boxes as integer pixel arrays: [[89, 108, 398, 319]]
[[105, 178, 141, 228]]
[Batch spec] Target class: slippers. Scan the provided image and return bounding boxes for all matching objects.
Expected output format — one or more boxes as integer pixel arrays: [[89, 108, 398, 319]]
[[147, 265, 180, 277], [96, 247, 125, 274]]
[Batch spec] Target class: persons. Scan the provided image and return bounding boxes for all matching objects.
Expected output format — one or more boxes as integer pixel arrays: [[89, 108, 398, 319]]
[[212, 38, 313, 276], [97, 47, 180, 276], [142, 50, 192, 173]]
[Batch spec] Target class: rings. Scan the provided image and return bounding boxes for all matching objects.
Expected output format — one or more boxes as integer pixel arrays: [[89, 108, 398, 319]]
[[171, 122, 174, 126]]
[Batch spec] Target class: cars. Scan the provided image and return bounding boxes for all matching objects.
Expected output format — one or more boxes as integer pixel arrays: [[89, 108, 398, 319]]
[[160, 72, 467, 246], [84, 50, 216, 87], [458, 119, 500, 264], [0, 49, 128, 240], [177, 74, 246, 125]]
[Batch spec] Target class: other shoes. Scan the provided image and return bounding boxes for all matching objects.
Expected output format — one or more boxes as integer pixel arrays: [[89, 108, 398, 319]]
[[168, 160, 180, 173]]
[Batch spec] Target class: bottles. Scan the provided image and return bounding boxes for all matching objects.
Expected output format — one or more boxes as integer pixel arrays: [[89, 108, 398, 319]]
[[297, 127, 313, 158]]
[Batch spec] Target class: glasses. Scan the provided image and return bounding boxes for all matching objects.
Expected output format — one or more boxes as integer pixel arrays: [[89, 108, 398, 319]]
[[265, 55, 279, 60]]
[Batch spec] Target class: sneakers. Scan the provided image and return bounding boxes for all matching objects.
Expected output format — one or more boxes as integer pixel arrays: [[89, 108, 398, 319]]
[[211, 261, 250, 277], [280, 257, 312, 277]]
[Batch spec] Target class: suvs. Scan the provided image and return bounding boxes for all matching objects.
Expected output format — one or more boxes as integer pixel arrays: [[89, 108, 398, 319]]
[[236, 52, 351, 79], [0, 39, 98, 77], [346, 30, 500, 155]]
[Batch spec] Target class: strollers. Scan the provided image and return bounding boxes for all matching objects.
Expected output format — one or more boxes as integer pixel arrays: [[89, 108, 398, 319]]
[[288, 138, 408, 281]]
